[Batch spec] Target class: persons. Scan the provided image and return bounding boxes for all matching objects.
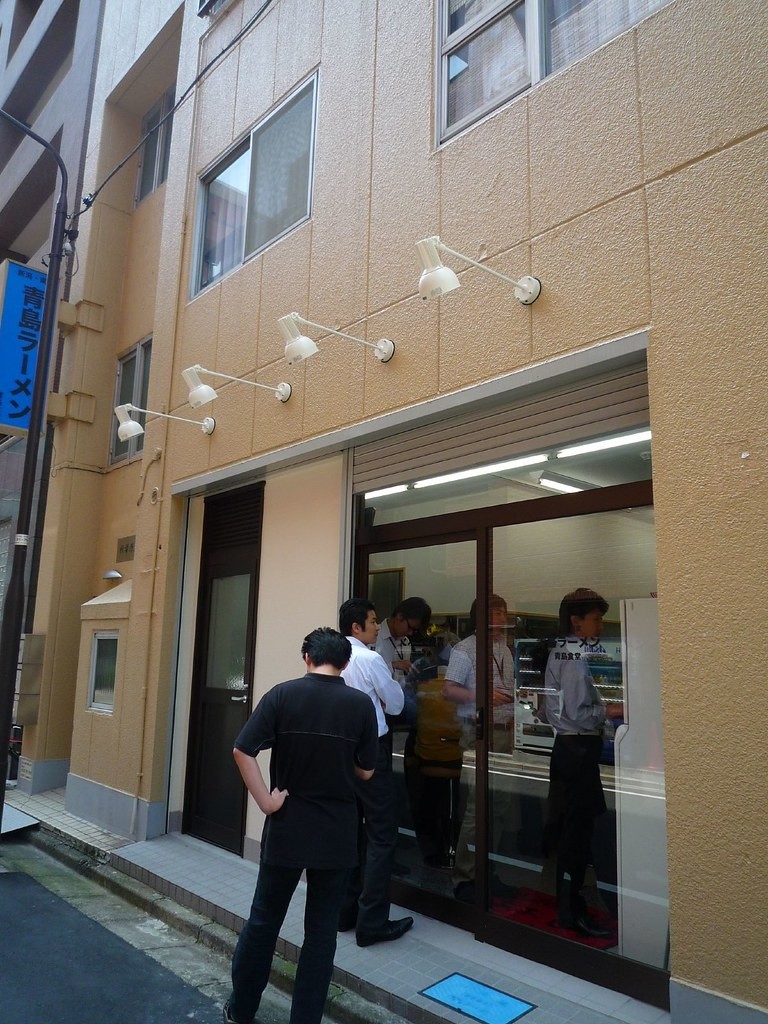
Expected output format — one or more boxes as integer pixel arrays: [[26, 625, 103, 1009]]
[[443, 594, 521, 910], [222, 627, 380, 1023], [545, 588, 618, 936], [367, 597, 432, 770], [338, 598, 413, 947], [402, 632, 465, 870]]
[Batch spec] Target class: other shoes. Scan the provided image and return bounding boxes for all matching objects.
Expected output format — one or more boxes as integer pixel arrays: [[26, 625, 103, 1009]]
[[487, 876, 518, 899], [391, 863, 411, 874], [223, 999, 240, 1024], [423, 854, 455, 869], [455, 883, 492, 907]]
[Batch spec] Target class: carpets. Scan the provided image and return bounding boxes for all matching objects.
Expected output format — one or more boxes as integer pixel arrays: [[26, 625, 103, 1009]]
[[493, 886, 618, 949]]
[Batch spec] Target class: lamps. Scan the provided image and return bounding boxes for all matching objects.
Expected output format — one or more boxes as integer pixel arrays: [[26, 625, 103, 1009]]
[[414, 236, 542, 305], [364, 484, 411, 500], [277, 311, 395, 365], [181, 364, 292, 410], [529, 470, 632, 514], [410, 449, 554, 489], [552, 427, 651, 460], [114, 402, 216, 442]]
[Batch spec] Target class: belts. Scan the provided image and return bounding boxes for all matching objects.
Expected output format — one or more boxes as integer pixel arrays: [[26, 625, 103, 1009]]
[[558, 730, 601, 735], [464, 717, 512, 731]]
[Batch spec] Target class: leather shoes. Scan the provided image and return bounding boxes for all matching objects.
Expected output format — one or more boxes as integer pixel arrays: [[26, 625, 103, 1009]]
[[338, 920, 356, 932], [357, 917, 413, 947], [559, 916, 613, 939]]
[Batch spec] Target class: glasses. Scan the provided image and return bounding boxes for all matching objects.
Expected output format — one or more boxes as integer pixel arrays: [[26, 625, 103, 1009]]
[[403, 615, 417, 632]]
